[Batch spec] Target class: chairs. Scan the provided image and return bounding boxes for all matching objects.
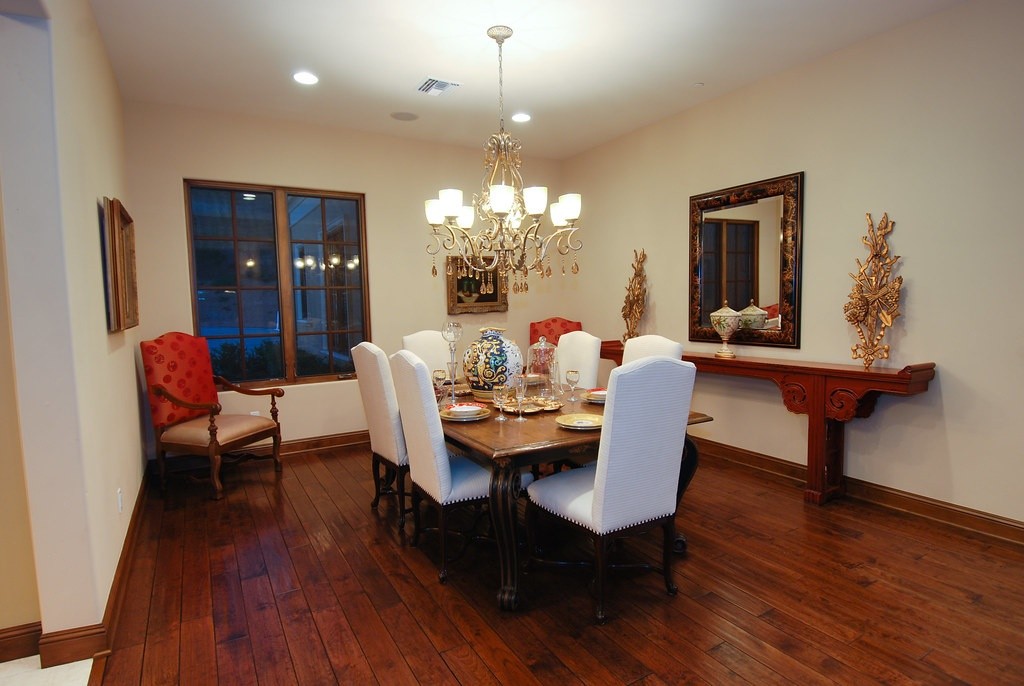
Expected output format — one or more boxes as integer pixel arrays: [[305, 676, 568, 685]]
[[530, 317, 582, 374], [350, 331, 701, 623], [136, 331, 282, 501]]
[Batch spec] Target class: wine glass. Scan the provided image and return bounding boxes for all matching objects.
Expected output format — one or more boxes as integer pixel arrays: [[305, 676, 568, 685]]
[[441, 322, 463, 408], [446, 363, 459, 400], [566, 371, 579, 402], [433, 369, 448, 411], [513, 376, 528, 423], [492, 386, 508, 423]]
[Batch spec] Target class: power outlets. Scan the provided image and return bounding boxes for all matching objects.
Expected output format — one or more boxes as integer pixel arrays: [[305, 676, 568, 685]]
[[250, 411, 257, 416], [117, 488, 123, 516]]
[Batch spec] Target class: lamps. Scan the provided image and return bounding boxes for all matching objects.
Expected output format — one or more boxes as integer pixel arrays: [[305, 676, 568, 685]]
[[424, 24, 582, 294]]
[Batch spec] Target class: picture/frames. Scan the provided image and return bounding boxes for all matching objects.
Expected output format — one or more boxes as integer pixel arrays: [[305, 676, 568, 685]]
[[447, 255, 507, 316], [97, 195, 136, 333]]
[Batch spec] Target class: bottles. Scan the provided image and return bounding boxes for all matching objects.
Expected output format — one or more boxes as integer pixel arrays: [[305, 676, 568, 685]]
[[527, 336, 558, 402], [710, 300, 742, 359], [739, 299, 768, 330], [463, 326, 523, 403]]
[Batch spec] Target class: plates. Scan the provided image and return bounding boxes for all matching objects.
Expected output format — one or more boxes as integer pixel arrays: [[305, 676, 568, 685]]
[[439, 372, 607, 430]]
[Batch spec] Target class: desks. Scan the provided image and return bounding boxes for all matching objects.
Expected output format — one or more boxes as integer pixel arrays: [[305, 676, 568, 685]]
[[426, 381, 714, 609], [590, 334, 936, 507]]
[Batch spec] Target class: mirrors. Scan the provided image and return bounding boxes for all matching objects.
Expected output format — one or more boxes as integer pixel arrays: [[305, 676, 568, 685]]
[[689, 173, 804, 342]]
